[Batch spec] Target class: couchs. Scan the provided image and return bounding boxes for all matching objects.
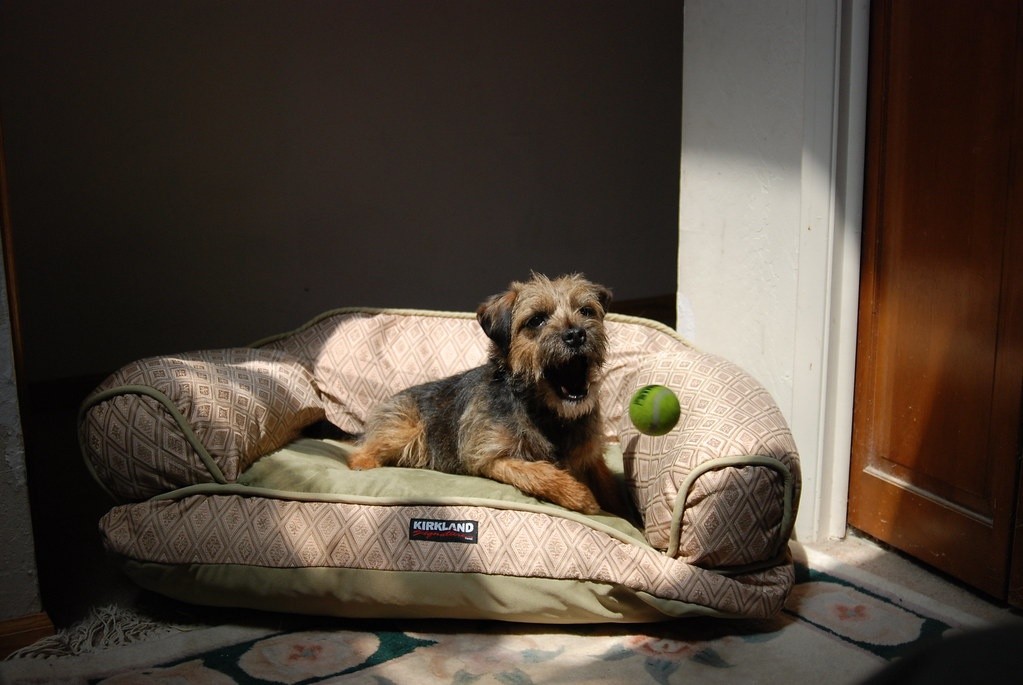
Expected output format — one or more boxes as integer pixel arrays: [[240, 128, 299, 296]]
[[74, 308, 803, 627]]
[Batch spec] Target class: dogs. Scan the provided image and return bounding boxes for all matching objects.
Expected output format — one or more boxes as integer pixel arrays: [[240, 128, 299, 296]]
[[348, 270, 644, 529]]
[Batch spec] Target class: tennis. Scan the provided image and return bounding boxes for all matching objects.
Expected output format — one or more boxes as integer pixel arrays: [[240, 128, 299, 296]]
[[627, 385, 680, 436]]
